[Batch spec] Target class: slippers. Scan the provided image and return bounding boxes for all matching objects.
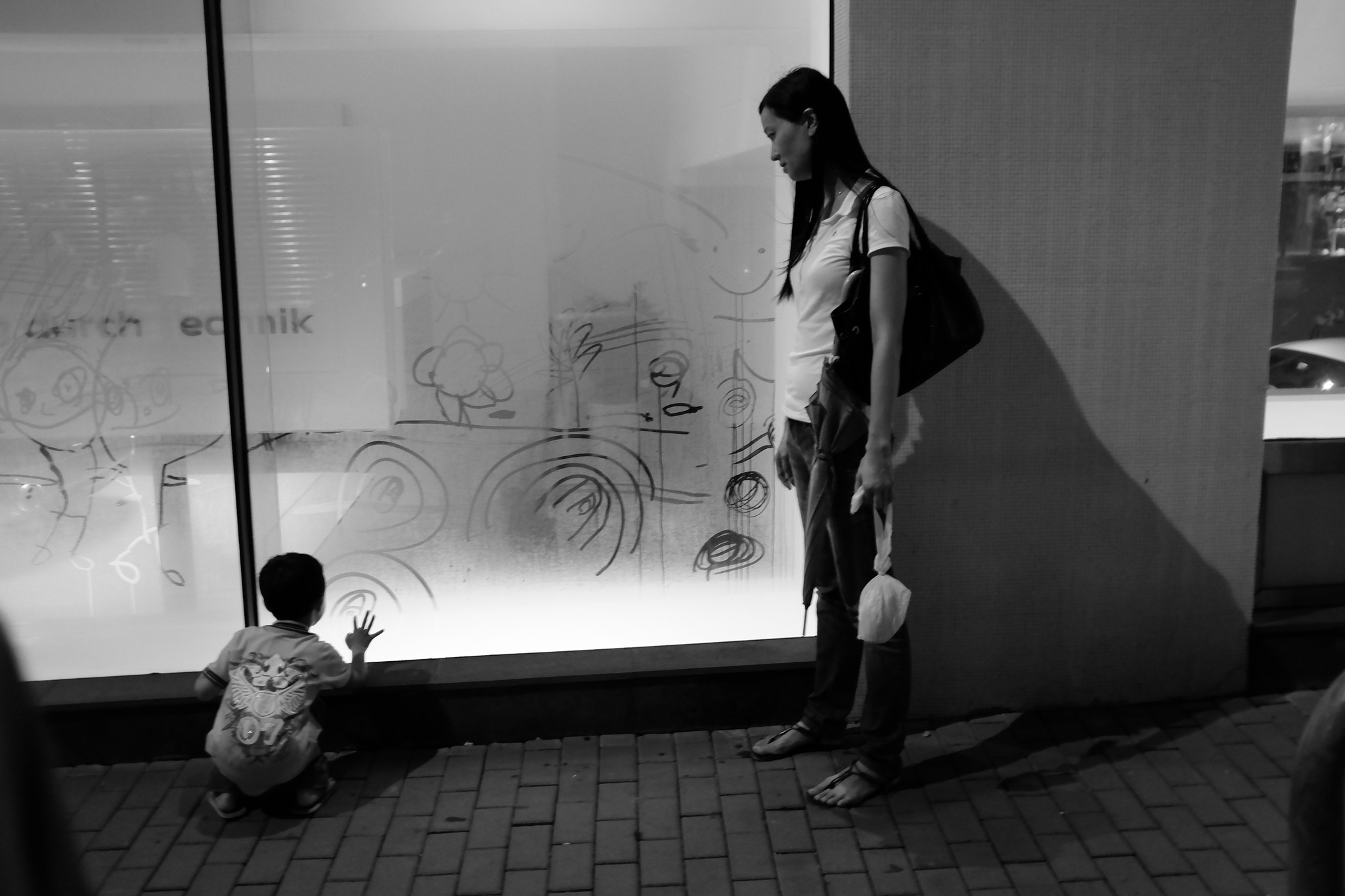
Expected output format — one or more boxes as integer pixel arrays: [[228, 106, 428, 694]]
[[812, 760, 903, 808], [297, 777, 337, 813], [750, 723, 841, 762], [207, 790, 246, 819]]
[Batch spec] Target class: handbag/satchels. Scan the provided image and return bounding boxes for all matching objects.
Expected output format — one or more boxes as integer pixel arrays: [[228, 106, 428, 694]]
[[830, 181, 984, 406]]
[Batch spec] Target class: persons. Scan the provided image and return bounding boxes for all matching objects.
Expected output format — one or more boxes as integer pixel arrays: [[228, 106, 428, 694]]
[[194, 552, 384, 819], [751, 66, 912, 807]]
